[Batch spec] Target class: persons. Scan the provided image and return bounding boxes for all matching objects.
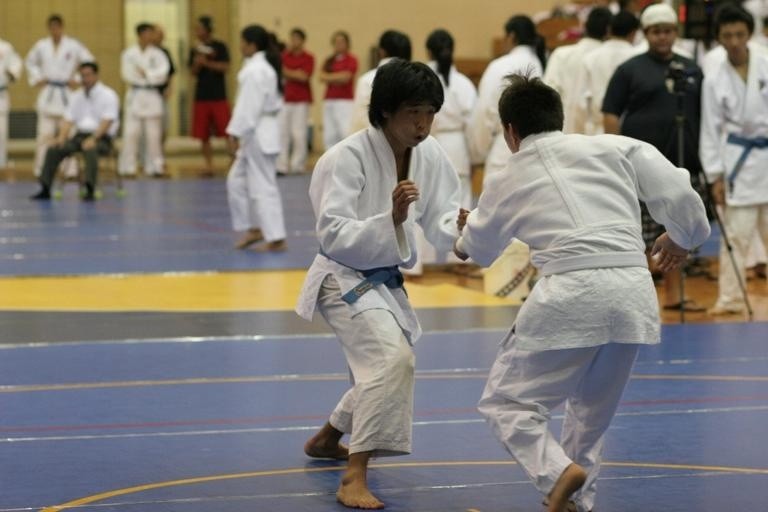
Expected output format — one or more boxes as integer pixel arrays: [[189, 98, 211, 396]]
[[356, 0, 768, 315], [293, 57, 471, 509], [0, 14, 358, 252], [452, 63, 711, 512]]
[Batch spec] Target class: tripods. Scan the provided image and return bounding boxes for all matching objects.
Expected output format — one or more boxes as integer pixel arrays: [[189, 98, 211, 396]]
[[662, 94, 756, 323]]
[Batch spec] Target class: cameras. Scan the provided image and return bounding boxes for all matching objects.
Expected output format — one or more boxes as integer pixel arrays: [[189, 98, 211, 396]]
[[670, 63, 687, 94]]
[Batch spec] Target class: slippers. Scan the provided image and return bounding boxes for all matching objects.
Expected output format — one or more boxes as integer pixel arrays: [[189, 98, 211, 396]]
[[663, 299, 706, 312]]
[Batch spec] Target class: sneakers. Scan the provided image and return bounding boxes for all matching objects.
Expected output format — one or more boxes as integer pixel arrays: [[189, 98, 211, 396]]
[[83, 192, 94, 201], [30, 192, 51, 199], [684, 260, 710, 275]]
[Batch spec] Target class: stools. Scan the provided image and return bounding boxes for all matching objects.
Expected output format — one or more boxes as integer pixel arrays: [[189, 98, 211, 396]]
[[56, 148, 126, 199]]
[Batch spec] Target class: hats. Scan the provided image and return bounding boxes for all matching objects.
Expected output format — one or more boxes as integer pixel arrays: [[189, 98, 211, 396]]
[[641, 4, 678, 28]]
